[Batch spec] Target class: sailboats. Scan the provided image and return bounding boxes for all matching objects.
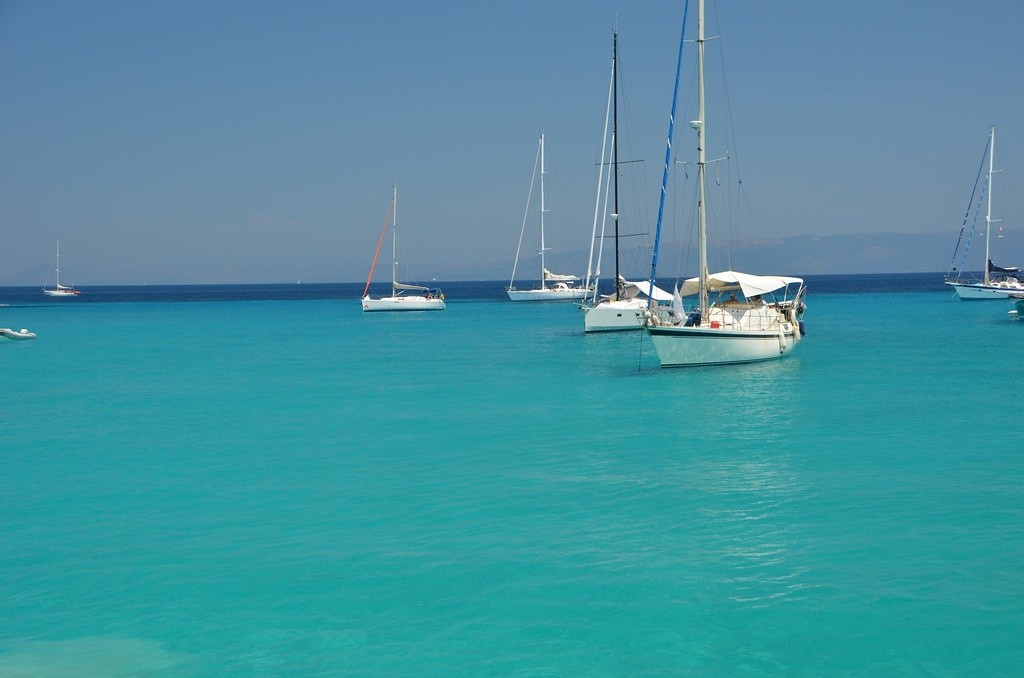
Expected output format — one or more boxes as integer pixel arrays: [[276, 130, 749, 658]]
[[573, 8, 677, 331], [637, 0, 808, 368], [42, 241, 80, 297], [943, 125, 1024, 300], [506, 133, 598, 301], [362, 185, 446, 311]]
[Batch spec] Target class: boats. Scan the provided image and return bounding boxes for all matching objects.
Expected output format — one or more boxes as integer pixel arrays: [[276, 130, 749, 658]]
[[0, 328, 36, 344]]
